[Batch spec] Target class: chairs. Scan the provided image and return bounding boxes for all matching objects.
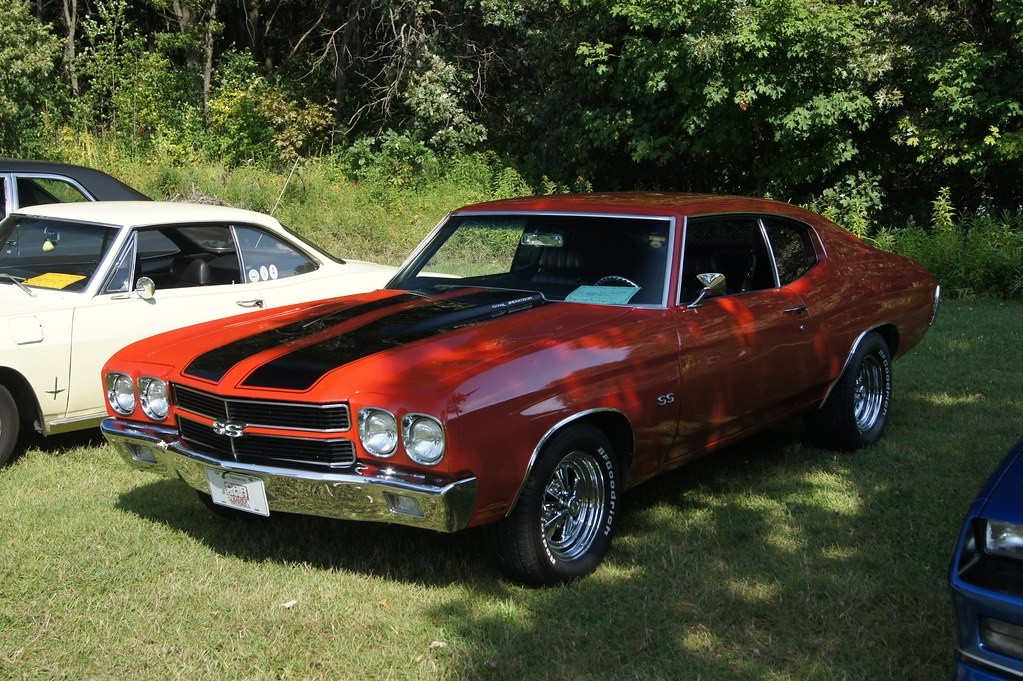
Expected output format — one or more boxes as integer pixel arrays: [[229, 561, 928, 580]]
[[528, 246, 586, 301], [169, 257, 211, 287]]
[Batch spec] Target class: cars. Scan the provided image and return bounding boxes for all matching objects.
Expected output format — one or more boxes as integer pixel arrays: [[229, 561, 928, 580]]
[[101, 191, 940, 591], [0, 201, 465, 471], [0, 157, 280, 269]]
